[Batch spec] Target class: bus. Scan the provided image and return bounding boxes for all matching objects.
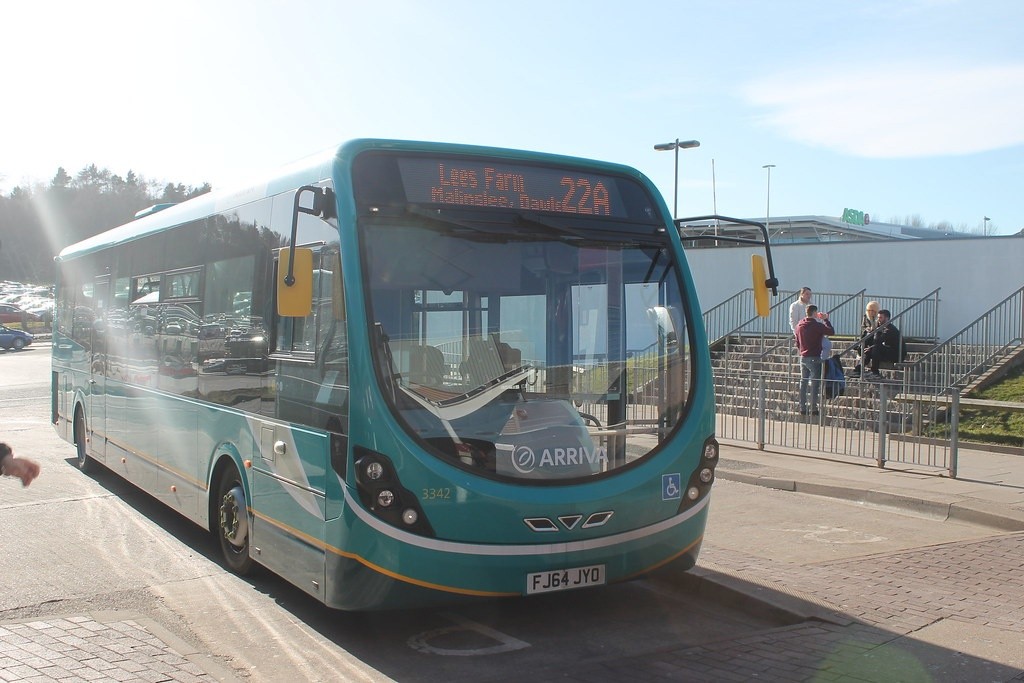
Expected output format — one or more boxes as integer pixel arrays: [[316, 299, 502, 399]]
[[48, 137, 779, 616]]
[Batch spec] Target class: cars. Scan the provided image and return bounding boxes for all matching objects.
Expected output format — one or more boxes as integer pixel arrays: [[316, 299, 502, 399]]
[[0, 280, 55, 322], [0, 325, 33, 350]]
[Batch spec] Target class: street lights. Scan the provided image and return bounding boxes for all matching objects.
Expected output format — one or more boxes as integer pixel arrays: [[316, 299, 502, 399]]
[[653, 138, 701, 219], [763, 165, 776, 239], [983, 216, 990, 236]]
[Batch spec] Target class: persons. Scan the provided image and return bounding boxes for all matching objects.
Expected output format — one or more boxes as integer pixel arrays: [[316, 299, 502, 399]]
[[849, 302, 880, 368], [795, 304, 835, 416], [854, 310, 907, 380], [790, 286, 831, 361], [0, 444, 40, 486]]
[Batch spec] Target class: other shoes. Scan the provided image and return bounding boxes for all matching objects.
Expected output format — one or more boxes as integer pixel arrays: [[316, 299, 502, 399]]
[[800, 411, 809, 416], [860, 372, 881, 380], [848, 370, 861, 377], [812, 411, 819, 416]]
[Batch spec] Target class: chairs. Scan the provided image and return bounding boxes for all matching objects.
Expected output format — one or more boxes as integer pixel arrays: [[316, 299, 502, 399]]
[[408, 345, 446, 387], [467, 343, 521, 384]]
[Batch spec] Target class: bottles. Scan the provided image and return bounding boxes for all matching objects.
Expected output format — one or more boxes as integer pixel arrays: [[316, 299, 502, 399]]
[[817, 313, 829, 319]]
